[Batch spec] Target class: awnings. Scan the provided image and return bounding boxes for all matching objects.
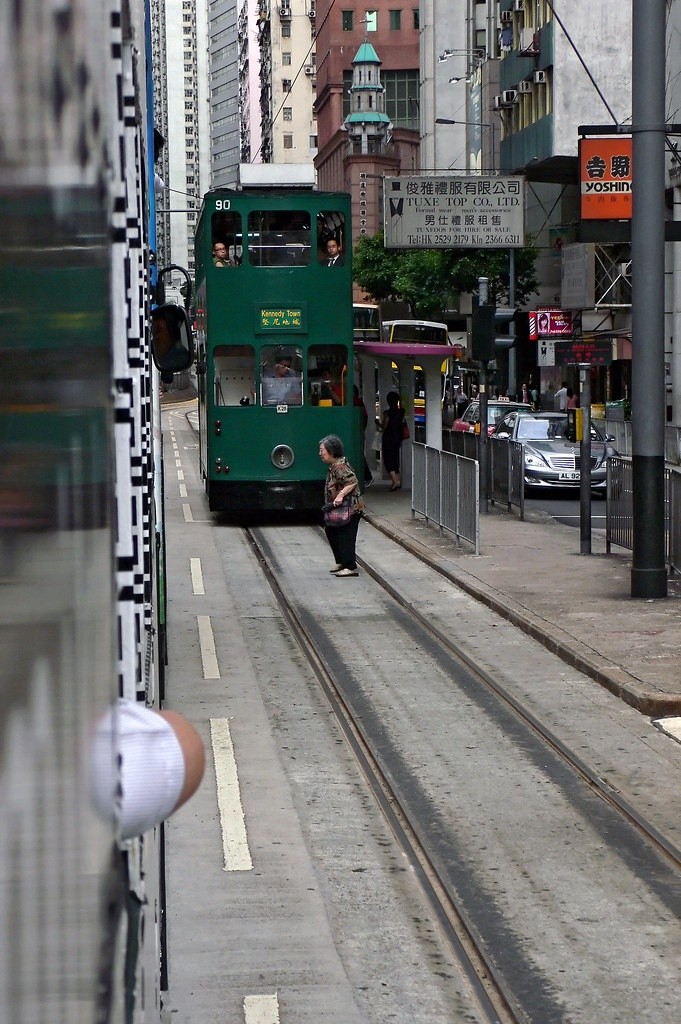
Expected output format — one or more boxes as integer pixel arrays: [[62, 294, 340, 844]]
[[547, 218, 681, 249], [512, 155, 578, 185], [589, 327, 632, 342]]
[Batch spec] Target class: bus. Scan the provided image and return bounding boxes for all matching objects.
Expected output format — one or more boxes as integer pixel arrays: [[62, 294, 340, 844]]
[[193, 187, 365, 512], [382, 320, 449, 423], [352, 303, 383, 416]]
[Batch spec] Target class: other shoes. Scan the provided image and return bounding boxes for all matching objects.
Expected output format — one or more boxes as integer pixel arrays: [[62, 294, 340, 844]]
[[390, 480, 401, 491], [365, 479, 375, 487], [329, 566, 360, 578]]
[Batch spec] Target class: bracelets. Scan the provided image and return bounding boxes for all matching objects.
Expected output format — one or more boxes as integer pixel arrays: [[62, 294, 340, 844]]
[[329, 388, 334, 392]]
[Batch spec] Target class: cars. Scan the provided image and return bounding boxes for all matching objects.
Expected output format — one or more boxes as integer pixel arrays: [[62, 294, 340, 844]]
[[451, 399, 536, 436], [491, 410, 623, 499]]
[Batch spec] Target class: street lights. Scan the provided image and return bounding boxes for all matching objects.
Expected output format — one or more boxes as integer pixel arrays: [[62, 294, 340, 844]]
[[435, 119, 517, 401]]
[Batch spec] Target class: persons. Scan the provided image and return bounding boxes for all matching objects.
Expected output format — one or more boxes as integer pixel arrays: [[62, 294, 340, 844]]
[[212, 216, 345, 266], [150, 305, 188, 366], [328, 373, 375, 488], [262, 357, 302, 405], [539, 313, 548, 332], [117, 697, 204, 843], [319, 436, 364, 577], [375, 391, 409, 491], [554, 381, 578, 411], [443, 381, 538, 418]]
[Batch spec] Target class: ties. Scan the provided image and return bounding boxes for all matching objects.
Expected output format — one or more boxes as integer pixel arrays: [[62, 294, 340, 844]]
[[330, 259, 335, 266]]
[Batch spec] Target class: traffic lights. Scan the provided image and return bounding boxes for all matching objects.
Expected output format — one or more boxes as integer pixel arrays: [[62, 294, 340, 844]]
[[471, 305, 519, 360]]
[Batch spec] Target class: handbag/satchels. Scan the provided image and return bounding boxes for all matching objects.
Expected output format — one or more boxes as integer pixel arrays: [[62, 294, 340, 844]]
[[324, 506, 351, 525], [403, 421, 411, 440]]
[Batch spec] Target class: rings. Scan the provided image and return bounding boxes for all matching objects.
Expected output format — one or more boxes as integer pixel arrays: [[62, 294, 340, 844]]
[[339, 503, 340, 504]]
